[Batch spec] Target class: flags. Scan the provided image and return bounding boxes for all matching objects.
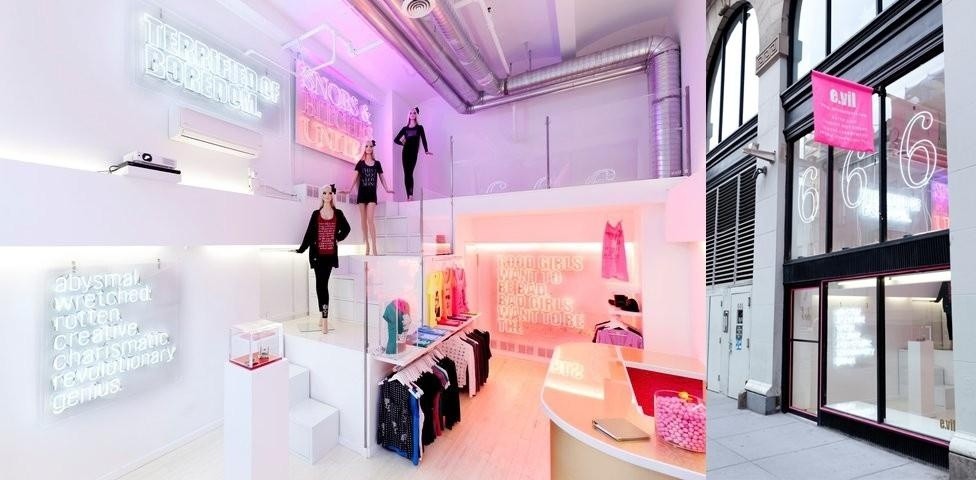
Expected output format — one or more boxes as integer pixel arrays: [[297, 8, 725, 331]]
[[809, 67, 875, 155]]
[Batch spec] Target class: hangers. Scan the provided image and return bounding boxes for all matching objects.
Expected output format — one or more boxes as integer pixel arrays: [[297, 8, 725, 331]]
[[378, 326, 479, 399], [595, 320, 633, 332]]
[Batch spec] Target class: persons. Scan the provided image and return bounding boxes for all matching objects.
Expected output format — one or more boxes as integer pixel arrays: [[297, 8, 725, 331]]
[[935, 282, 952, 352], [391, 104, 433, 200]]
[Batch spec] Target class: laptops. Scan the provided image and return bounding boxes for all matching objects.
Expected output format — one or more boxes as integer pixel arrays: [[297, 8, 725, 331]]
[[592, 418, 650, 441]]
